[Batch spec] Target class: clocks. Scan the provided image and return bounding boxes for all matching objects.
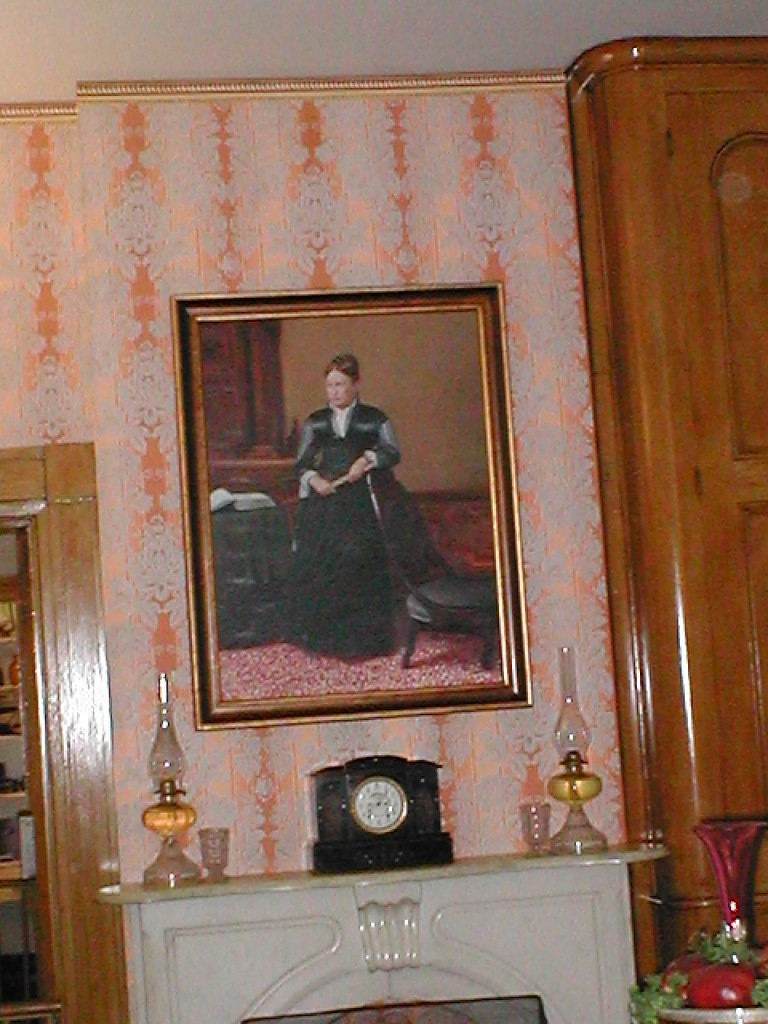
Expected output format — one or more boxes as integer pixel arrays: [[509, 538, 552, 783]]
[[307, 756, 454, 875]]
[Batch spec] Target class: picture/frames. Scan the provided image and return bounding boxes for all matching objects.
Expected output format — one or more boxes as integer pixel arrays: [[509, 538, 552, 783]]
[[169, 276, 535, 734]]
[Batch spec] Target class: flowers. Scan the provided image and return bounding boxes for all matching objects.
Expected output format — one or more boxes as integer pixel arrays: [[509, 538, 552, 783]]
[[628, 920, 768, 1024]]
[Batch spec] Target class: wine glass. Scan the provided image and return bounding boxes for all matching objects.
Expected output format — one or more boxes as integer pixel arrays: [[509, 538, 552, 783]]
[[519, 803, 555, 859], [198, 827, 233, 885]]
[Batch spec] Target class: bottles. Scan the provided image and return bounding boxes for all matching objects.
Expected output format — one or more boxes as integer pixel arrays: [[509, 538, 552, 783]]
[[9, 654, 21, 686]]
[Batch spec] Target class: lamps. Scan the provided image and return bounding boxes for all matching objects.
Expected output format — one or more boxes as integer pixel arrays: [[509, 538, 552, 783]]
[[143, 672, 202, 884], [548, 647, 608, 854]]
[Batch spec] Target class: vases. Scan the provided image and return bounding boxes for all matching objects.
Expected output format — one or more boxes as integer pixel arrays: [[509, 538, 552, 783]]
[[657, 1007, 768, 1024]]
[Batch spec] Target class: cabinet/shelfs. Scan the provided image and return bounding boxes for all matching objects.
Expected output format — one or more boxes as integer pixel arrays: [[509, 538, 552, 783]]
[[0, 636, 37, 1001]]
